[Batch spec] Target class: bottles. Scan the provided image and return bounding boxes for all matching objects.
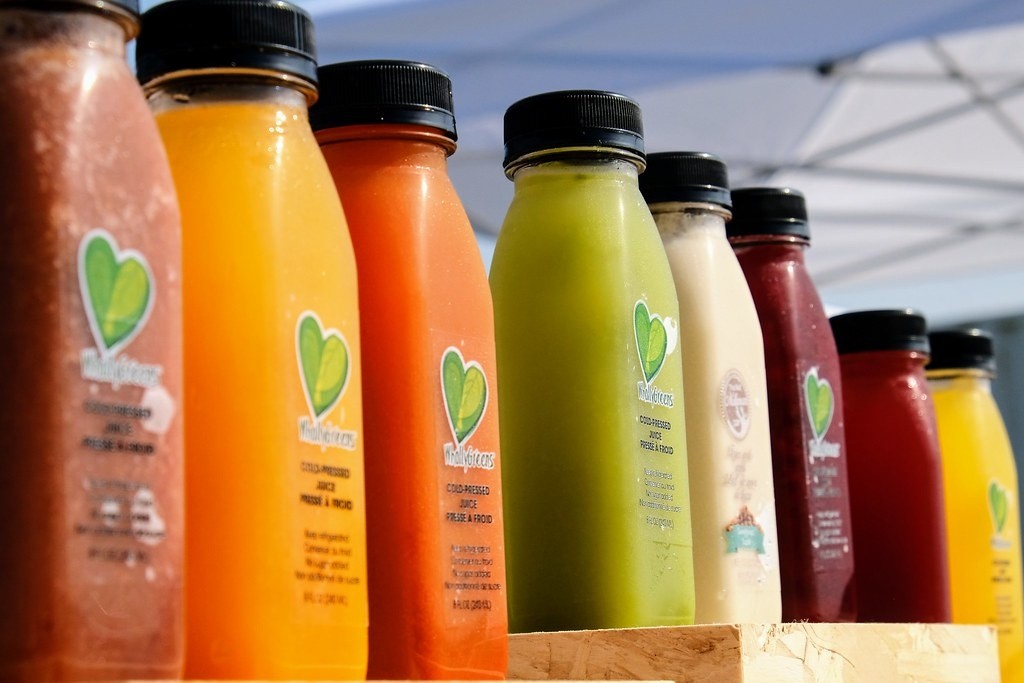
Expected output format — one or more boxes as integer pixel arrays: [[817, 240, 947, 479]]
[[305, 59, 510, 682], [829, 310, 952, 623], [725, 186, 859, 622], [484, 89, 697, 635], [922, 329, 1024, 683], [131, 0, 370, 681], [0, 0, 186, 683], [638, 152, 783, 624]]
